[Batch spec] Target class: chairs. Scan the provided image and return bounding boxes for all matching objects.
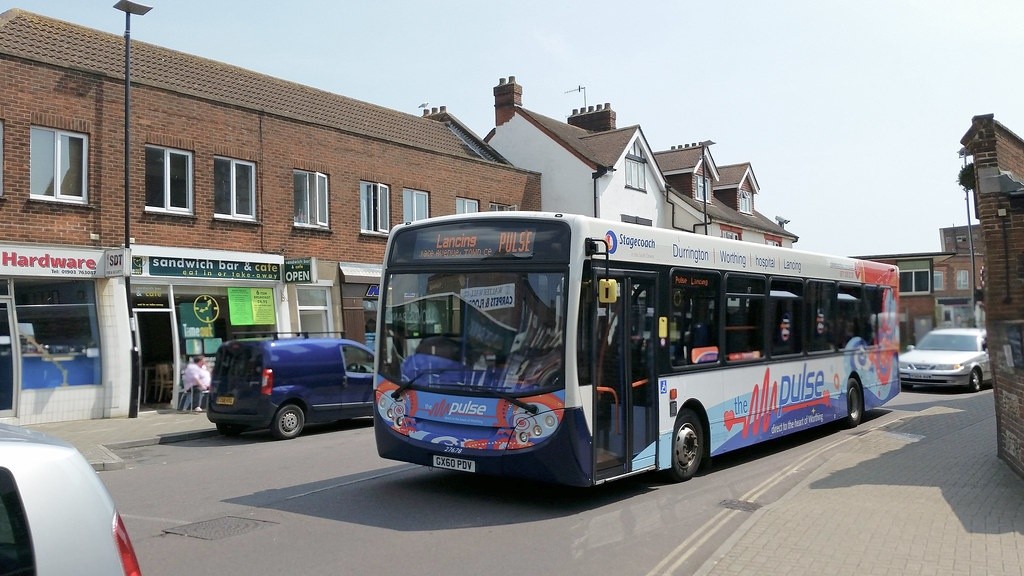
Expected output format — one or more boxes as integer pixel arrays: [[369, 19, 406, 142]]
[[158, 364, 173, 402], [154, 364, 172, 402]]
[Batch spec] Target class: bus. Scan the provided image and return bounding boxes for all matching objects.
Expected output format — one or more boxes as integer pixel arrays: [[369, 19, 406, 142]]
[[386, 293, 517, 374], [372, 211, 902, 487]]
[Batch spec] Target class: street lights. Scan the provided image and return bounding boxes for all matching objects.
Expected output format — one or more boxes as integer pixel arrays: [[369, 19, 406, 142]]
[[112, 1, 154, 420], [699, 141, 717, 236], [958, 148, 975, 326]]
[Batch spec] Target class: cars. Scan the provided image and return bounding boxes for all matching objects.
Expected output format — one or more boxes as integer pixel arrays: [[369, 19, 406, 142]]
[[899, 327, 993, 392], [0, 423, 143, 576]]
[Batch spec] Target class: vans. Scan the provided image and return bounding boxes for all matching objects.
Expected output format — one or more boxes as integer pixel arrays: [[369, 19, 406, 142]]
[[207, 333, 405, 439]]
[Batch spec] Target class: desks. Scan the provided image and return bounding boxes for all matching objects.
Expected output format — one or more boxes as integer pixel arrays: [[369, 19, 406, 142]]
[[143, 366, 156, 405]]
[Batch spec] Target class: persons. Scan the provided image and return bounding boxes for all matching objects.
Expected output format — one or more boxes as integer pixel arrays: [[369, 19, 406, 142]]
[[182, 355, 211, 414]]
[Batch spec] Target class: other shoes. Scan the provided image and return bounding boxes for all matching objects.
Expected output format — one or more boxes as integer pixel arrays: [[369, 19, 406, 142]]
[[195, 407, 202, 412], [176, 410, 188, 414]]
